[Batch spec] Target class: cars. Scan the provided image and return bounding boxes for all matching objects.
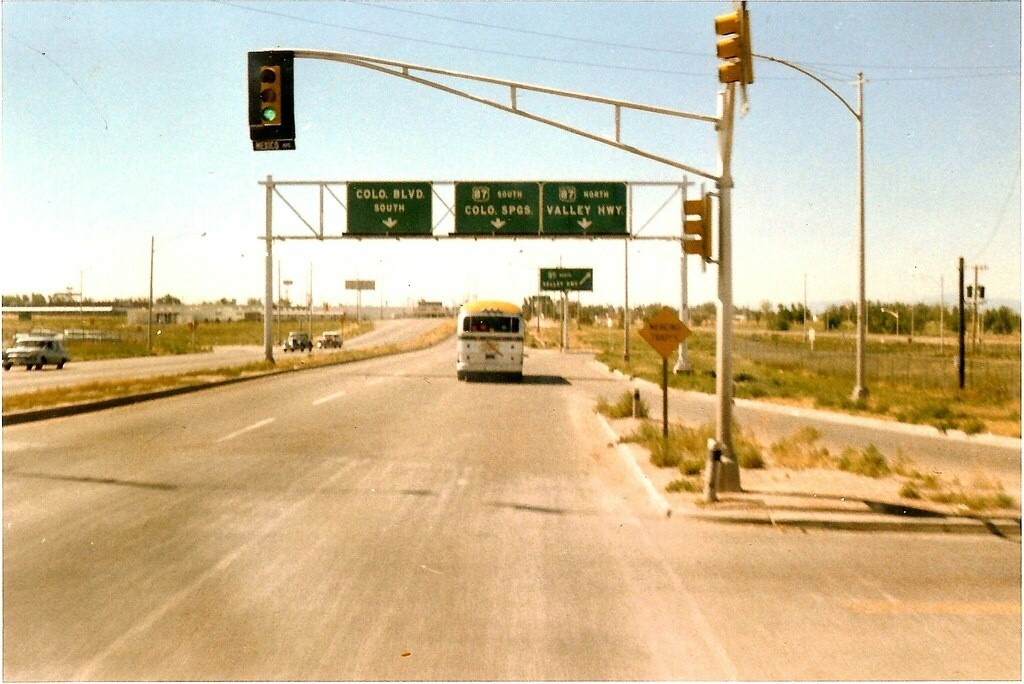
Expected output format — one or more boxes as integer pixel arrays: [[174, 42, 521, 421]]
[[318, 331, 343, 349], [280, 332, 313, 352], [2, 336, 71, 373]]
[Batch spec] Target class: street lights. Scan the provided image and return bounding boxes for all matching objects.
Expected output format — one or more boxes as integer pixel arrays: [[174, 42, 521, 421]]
[[879, 305, 900, 337]]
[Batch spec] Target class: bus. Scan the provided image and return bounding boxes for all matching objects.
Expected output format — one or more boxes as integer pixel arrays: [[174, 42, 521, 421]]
[[454, 300, 527, 382]]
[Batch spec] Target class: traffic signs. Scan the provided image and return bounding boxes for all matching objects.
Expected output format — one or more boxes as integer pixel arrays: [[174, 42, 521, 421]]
[[543, 180, 626, 234], [455, 181, 540, 235], [346, 181, 432, 236], [540, 268, 592, 291]]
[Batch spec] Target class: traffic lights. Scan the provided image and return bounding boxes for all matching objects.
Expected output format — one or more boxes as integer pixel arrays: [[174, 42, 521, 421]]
[[247, 50, 294, 140], [715, 10, 754, 87], [682, 194, 715, 257]]
